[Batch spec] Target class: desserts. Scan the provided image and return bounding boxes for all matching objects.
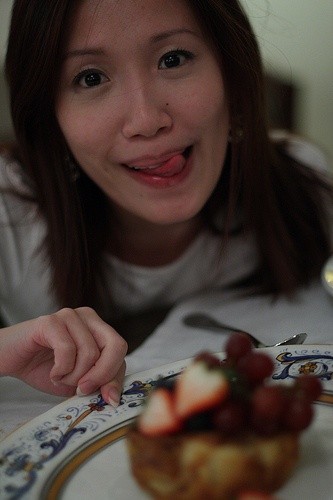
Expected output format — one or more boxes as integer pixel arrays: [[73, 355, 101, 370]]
[[129, 332, 322, 500]]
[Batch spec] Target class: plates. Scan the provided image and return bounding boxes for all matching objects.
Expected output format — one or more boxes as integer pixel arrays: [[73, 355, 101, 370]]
[[1, 345, 333, 500], [322, 258, 333, 296]]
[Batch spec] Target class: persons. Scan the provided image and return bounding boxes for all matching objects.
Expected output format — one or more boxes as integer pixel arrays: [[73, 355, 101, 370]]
[[1, 0, 333, 409]]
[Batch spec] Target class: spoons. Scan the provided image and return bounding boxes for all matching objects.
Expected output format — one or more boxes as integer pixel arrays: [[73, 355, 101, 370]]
[[182, 312, 307, 347]]
